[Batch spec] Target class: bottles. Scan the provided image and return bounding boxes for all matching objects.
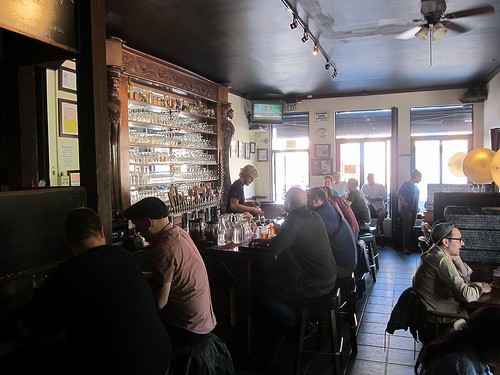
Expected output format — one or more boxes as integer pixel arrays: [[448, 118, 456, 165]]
[[188, 180, 219, 204], [127, 83, 217, 118], [183, 207, 259, 247]]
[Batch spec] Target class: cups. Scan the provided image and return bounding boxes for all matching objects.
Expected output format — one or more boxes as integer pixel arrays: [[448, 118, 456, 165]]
[[260, 210, 288, 239]]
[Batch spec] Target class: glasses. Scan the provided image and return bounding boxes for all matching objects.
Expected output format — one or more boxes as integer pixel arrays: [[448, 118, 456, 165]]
[[447, 236, 462, 243]]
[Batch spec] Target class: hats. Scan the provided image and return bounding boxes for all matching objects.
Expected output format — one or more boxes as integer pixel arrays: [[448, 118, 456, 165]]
[[429, 222, 454, 251], [124, 197, 168, 220]]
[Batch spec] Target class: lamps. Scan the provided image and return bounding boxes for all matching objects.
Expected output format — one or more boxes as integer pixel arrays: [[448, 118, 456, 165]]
[[313, 42, 318, 55], [324, 58, 330, 71], [290, 14, 298, 29], [302, 28, 309, 43], [331, 68, 337, 78], [415, 20, 448, 41]]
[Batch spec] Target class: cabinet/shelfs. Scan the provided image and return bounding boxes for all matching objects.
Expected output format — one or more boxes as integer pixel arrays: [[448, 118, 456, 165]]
[[106, 36, 234, 248]]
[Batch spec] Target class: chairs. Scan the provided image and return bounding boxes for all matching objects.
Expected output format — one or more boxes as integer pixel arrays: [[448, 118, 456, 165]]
[[384, 287, 469, 375]]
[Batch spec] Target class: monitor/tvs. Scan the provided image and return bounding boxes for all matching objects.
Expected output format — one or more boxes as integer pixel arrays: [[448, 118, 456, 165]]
[[251, 100, 285, 124]]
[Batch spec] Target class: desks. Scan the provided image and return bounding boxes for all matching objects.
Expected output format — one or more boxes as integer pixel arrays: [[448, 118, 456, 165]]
[[476, 293, 500, 308]]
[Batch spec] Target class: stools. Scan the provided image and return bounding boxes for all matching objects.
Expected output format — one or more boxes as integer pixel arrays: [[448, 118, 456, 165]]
[[165, 328, 216, 375], [298, 226, 380, 375]]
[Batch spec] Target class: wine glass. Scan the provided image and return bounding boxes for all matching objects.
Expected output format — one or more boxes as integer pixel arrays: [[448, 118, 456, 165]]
[[127, 107, 218, 181], [130, 183, 193, 208]]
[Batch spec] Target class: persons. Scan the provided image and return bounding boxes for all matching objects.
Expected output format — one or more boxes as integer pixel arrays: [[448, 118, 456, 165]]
[[397, 169, 422, 255], [226, 164, 264, 217], [361, 173, 387, 237], [248, 187, 338, 340], [411, 220, 491, 324], [122, 196, 217, 348], [305, 171, 371, 279], [21, 207, 171, 375], [414, 305, 500, 375]]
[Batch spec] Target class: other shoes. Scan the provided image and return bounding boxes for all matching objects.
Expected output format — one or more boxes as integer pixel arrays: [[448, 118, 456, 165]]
[[280, 321, 316, 351], [404, 248, 409, 254]]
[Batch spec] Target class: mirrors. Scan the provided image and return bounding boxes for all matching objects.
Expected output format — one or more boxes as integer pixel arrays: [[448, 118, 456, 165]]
[[0, 23, 86, 197]]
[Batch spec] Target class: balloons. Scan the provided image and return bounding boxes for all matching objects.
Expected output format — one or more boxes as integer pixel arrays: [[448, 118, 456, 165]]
[[448, 152, 466, 175], [463, 148, 500, 188]]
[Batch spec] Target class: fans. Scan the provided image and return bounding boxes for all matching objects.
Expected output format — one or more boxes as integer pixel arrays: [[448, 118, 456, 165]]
[[379, 0, 495, 38]]
